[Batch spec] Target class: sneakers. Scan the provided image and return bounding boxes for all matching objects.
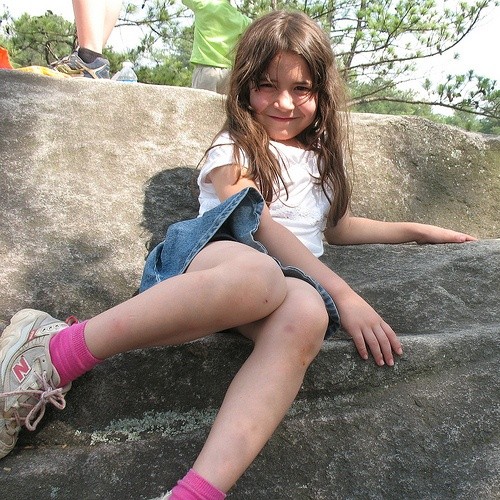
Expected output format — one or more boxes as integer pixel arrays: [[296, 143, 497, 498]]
[[0, 309, 80, 460], [48, 51, 111, 80]]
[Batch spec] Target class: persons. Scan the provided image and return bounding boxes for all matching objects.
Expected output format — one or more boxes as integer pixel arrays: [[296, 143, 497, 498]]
[[0, 11, 478, 500], [181, 0, 254, 95], [46, 0, 123, 80]]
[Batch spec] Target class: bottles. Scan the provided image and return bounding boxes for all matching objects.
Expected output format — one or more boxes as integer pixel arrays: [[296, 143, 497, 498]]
[[116, 61, 138, 82]]
